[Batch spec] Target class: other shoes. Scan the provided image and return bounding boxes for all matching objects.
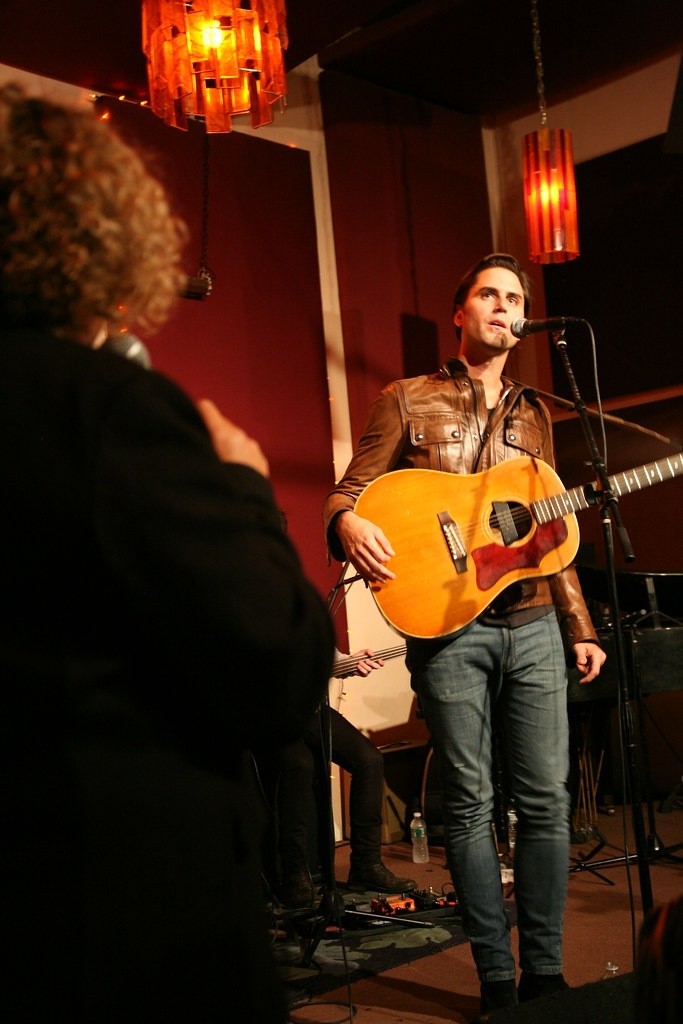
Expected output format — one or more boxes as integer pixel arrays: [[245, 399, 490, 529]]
[[479, 988, 518, 1024], [346, 860, 417, 893], [288, 868, 322, 908]]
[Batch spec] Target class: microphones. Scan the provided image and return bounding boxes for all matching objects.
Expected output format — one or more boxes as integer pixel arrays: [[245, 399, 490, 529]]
[[511, 316, 581, 341]]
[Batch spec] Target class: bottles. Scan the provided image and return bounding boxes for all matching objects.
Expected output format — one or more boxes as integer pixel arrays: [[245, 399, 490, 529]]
[[506, 798, 518, 849], [411, 812, 430, 865], [601, 961, 619, 979]]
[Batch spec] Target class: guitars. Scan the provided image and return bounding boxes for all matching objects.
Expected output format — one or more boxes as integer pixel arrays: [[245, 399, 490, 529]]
[[331, 643, 407, 677], [352, 453, 683, 643]]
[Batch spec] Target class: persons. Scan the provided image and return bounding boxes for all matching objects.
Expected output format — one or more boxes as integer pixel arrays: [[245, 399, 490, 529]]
[[323, 252, 607, 1024], [0, 93, 417, 1024]]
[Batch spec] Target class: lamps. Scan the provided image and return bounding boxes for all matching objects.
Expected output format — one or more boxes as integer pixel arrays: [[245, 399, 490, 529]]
[[141, 0, 289, 133], [522, 0, 583, 263]]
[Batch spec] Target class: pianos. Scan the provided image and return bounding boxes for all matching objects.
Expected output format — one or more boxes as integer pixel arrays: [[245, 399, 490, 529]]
[[488, 562, 683, 870]]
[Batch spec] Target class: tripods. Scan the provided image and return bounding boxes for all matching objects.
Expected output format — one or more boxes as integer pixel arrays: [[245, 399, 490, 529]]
[[270, 684, 438, 968], [580, 633, 683, 870]]
